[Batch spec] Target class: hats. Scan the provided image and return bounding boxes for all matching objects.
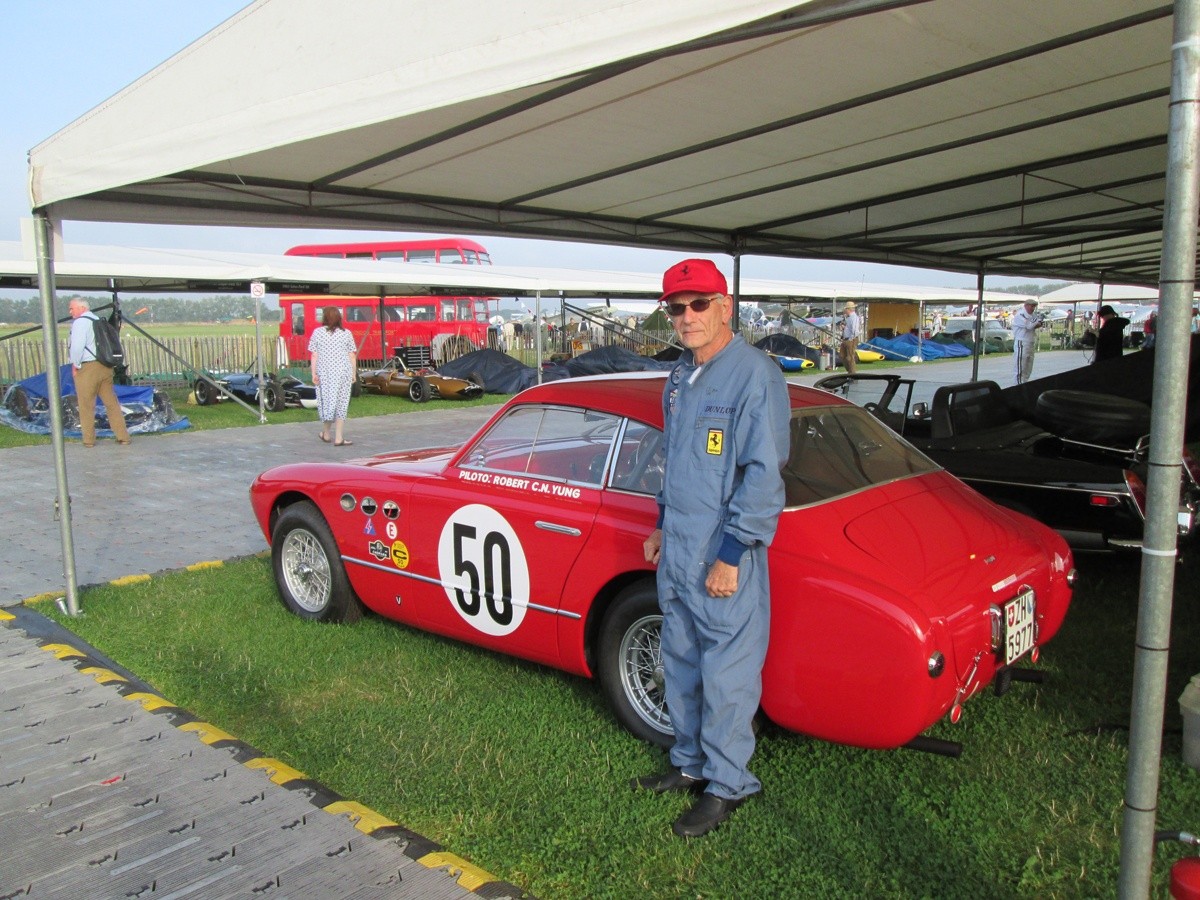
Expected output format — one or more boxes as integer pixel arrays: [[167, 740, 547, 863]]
[[658, 257, 727, 301], [844, 301, 857, 309], [1025, 298, 1038, 305]]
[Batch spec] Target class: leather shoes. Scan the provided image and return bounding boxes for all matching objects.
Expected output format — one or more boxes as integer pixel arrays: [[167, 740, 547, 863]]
[[630, 766, 703, 795], [674, 792, 744, 838]]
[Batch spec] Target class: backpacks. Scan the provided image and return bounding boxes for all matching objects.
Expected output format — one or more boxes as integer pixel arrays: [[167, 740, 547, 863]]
[[71, 316, 124, 369]]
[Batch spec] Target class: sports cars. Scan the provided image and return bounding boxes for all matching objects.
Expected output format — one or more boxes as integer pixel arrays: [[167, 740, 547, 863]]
[[193, 358, 486, 413], [252, 324, 1200, 761]]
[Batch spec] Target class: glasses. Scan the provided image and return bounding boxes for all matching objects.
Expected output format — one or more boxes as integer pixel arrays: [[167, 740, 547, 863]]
[[664, 296, 721, 316]]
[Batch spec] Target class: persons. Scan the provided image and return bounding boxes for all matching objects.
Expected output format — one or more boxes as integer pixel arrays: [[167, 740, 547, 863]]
[[1092, 304, 1130, 363], [1011, 299, 1042, 386], [839, 301, 861, 385], [1139, 307, 1200, 350], [68, 297, 131, 449], [638, 258, 792, 837], [490, 304, 1094, 357], [308, 306, 357, 447]]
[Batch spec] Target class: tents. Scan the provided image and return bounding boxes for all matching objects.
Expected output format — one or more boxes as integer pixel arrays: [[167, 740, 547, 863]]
[[27, 0, 1200, 900], [2, 236, 1047, 429], [1039, 282, 1200, 338]]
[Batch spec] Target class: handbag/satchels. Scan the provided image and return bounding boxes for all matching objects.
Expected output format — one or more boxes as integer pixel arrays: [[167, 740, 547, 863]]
[[1081, 329, 1097, 347]]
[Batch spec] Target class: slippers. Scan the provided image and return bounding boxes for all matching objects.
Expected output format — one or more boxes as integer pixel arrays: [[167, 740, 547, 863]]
[[334, 440, 353, 446], [319, 432, 331, 442]]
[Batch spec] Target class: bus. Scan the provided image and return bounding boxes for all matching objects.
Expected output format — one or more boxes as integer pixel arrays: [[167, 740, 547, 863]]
[[280, 237, 503, 364]]
